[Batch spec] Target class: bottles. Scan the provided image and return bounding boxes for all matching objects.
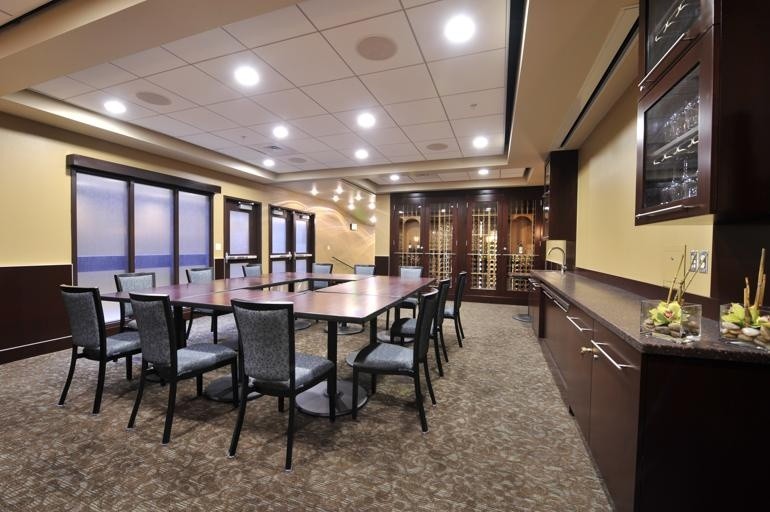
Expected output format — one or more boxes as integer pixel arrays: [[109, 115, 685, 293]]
[[408, 240, 412, 252], [518, 241, 524, 254], [417, 240, 421, 252]]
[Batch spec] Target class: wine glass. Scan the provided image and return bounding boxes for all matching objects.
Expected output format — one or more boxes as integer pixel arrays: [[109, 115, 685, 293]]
[[659, 155, 699, 204], [663, 75, 699, 144]]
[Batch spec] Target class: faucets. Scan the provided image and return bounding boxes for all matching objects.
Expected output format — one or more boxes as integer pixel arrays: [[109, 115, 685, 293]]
[[547, 247, 567, 272]]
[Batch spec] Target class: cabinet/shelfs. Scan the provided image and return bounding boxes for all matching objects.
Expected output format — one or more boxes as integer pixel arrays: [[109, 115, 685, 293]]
[[544, 149, 578, 239], [635, 0, 770, 227]]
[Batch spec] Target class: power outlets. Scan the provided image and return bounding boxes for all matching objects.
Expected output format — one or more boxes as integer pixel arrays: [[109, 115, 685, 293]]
[[689, 250, 708, 272]]
